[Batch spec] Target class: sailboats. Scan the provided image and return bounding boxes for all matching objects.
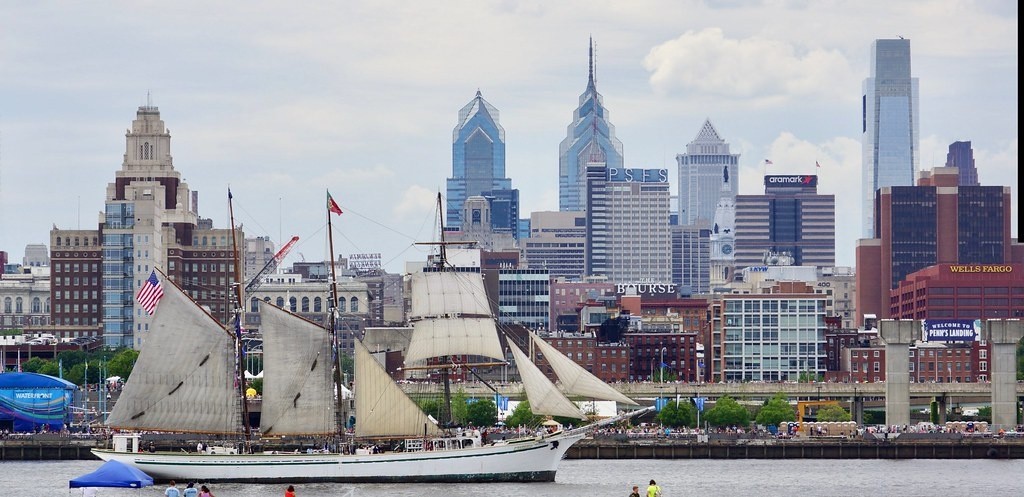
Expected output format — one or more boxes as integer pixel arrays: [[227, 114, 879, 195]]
[[89, 189, 656, 484]]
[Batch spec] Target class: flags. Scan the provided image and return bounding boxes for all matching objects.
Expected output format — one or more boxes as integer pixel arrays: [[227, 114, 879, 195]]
[[136, 269, 163, 316], [326, 190, 342, 216]]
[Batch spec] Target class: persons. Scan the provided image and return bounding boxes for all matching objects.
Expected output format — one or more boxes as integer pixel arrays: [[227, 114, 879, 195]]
[[556, 329, 597, 338], [646, 479, 660, 497], [910, 376, 989, 384], [396, 379, 521, 385], [454, 422, 578, 437], [839, 420, 1024, 443], [590, 423, 832, 442], [629, 485, 640, 497], [164, 481, 214, 497], [85, 381, 123, 392], [270, 447, 317, 455], [186, 441, 204, 454], [138, 441, 156, 453], [247, 394, 261, 399], [336, 442, 386, 454], [1018, 379, 1023, 383], [285, 485, 295, 497], [0, 422, 188, 438], [421, 440, 446, 450], [604, 377, 885, 384]]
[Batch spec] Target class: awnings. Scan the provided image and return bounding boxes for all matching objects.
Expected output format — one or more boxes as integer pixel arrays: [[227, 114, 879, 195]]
[[68, 459, 154, 497]]
